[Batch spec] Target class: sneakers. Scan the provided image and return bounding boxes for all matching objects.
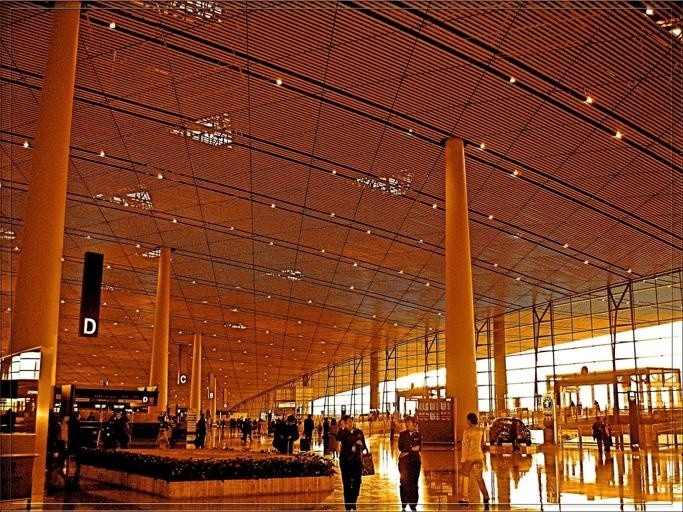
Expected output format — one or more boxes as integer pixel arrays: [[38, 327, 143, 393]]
[[458, 496, 489, 511]]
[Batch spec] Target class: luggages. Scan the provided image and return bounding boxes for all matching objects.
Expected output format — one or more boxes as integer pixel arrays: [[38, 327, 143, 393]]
[[299, 432, 311, 451]]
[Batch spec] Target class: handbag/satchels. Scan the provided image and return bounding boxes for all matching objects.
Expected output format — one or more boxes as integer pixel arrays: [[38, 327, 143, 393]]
[[481, 427, 487, 454], [358, 442, 375, 475]]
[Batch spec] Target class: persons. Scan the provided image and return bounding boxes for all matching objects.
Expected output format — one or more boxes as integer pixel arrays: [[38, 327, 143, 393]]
[[594, 400, 602, 416], [3, 409, 16, 432], [45, 402, 130, 492], [508, 417, 520, 453], [333, 413, 368, 512], [397, 415, 422, 512], [389, 417, 396, 442], [592, 417, 605, 455], [458, 411, 490, 507], [368, 410, 380, 422], [568, 401, 574, 407], [578, 402, 582, 407], [602, 418, 613, 454], [194, 412, 332, 456]]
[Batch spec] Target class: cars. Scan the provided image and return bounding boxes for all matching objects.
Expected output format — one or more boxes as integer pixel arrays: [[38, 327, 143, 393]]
[[489, 417, 531, 446]]
